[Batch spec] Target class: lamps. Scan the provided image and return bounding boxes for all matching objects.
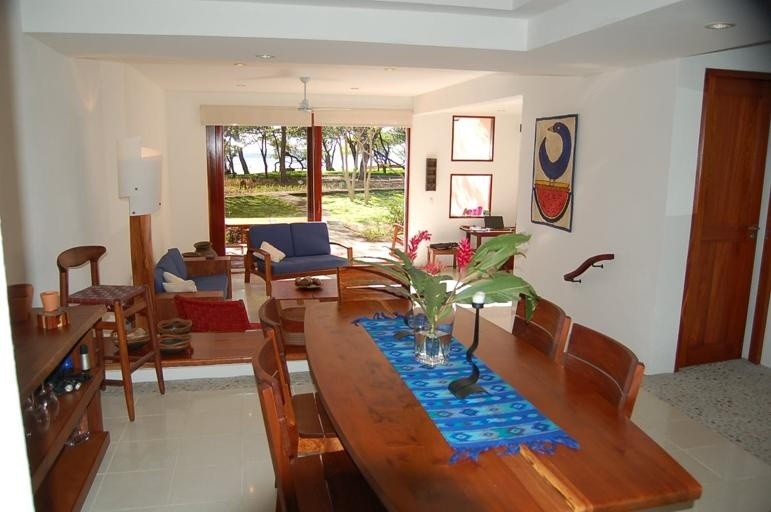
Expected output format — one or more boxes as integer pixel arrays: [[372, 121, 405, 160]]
[[114, 136, 163, 216]]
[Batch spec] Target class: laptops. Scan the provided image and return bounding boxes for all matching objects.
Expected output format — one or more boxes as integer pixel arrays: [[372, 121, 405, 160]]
[[484, 216, 509, 231]]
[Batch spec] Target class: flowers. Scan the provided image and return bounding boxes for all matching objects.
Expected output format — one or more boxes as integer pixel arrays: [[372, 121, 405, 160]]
[[349, 232, 540, 362]]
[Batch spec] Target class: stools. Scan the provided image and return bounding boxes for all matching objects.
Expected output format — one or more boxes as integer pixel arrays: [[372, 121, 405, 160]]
[[426, 247, 458, 269]]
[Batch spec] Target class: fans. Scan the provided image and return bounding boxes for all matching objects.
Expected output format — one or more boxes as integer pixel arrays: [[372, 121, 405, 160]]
[[264, 76, 352, 112]]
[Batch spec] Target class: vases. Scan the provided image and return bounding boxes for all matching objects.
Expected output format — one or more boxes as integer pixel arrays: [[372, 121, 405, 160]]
[[194, 241, 217, 256]]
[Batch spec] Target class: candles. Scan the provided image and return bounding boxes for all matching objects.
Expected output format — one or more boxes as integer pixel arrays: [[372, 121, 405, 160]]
[[472, 291, 485, 304]]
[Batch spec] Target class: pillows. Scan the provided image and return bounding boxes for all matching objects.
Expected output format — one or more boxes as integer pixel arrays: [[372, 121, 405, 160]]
[[154, 248, 197, 293], [248, 222, 331, 262]]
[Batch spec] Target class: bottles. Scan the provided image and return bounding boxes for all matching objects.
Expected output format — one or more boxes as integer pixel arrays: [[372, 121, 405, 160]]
[[78, 344, 93, 388]]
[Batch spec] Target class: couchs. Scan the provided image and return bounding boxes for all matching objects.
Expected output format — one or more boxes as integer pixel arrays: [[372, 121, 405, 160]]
[[242, 230, 353, 296], [151, 255, 232, 301]]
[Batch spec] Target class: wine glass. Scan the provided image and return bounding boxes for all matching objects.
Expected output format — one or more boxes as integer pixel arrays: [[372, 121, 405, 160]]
[[25, 381, 59, 436]]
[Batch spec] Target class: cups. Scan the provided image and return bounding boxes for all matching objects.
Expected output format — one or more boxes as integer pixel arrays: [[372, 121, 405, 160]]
[[8, 284, 60, 323]]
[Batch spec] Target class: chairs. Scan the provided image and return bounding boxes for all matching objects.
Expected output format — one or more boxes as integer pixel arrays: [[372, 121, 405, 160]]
[[561, 322, 645, 421], [57, 245, 166, 422], [337, 265, 412, 302], [511, 291, 572, 362], [258, 298, 345, 456], [251, 327, 385, 511], [389, 223, 405, 261]]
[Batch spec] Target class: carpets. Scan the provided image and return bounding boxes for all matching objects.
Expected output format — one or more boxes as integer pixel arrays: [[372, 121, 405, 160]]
[[639, 358, 771, 469]]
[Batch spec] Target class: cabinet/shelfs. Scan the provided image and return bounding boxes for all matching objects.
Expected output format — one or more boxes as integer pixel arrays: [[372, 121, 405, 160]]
[[9, 305, 111, 512]]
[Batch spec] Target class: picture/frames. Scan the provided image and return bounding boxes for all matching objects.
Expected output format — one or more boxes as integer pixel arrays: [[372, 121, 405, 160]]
[[449, 173, 493, 218], [451, 114, 495, 162]]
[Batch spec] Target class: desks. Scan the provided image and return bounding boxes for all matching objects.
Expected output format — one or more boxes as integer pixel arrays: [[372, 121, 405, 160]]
[[459, 225, 516, 270], [304, 301, 703, 512]]
